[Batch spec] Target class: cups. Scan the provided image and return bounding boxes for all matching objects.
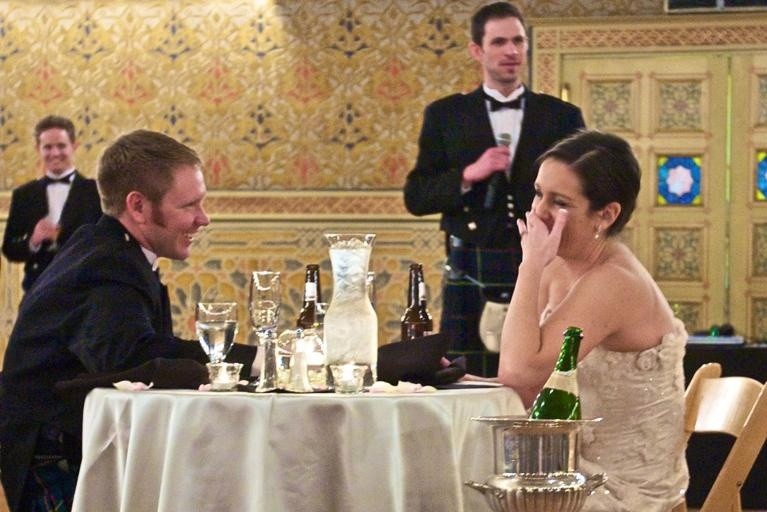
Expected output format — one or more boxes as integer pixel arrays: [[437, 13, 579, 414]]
[[329, 363, 369, 394], [205, 361, 245, 385]]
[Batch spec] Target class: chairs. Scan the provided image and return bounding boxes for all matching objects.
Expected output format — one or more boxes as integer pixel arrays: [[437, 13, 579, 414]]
[[685, 362, 767, 512]]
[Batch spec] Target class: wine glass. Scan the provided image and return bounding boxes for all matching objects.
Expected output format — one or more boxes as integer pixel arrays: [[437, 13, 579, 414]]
[[246, 269, 281, 393], [194, 300, 241, 363]]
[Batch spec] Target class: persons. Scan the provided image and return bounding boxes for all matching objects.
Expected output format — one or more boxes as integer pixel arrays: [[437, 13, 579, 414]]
[[1, 129, 265, 510], [441, 130, 690, 512], [1, 116, 103, 294], [403, 1, 586, 381]]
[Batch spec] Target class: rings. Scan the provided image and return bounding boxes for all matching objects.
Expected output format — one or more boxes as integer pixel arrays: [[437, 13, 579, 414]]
[[521, 230, 527, 238]]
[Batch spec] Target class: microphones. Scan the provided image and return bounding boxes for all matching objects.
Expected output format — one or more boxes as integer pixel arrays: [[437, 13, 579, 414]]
[[484, 133, 512, 209]]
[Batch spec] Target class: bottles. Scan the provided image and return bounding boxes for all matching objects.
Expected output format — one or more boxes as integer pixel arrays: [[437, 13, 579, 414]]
[[295, 263, 326, 330], [288, 339, 312, 392], [398, 264, 433, 342], [480, 132, 514, 211], [525, 326, 584, 420]]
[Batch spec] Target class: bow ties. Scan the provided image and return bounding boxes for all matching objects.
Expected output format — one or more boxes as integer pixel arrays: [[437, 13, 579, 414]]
[[484, 93, 526, 111], [45, 171, 75, 185]]
